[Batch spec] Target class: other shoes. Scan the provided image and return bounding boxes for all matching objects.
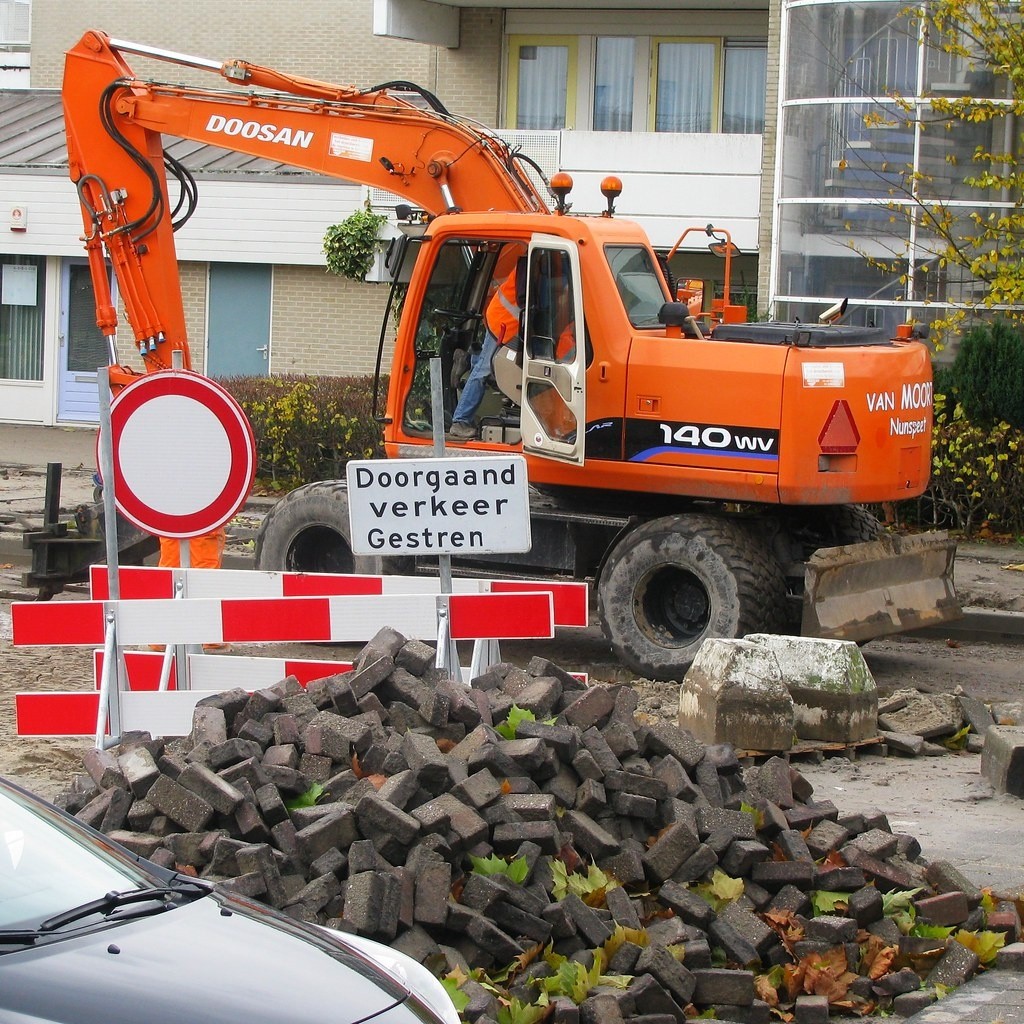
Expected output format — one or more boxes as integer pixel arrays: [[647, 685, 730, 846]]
[[450, 422, 476, 437]]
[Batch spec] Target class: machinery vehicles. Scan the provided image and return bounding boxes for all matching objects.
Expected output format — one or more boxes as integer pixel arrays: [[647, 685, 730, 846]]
[[63, 29, 967, 687]]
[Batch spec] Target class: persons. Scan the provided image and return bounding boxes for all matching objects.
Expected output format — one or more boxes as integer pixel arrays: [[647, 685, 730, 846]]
[[448, 254, 568, 438]]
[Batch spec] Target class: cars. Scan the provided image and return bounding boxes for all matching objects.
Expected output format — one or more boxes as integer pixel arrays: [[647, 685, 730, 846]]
[[2, 778, 462, 1024]]
